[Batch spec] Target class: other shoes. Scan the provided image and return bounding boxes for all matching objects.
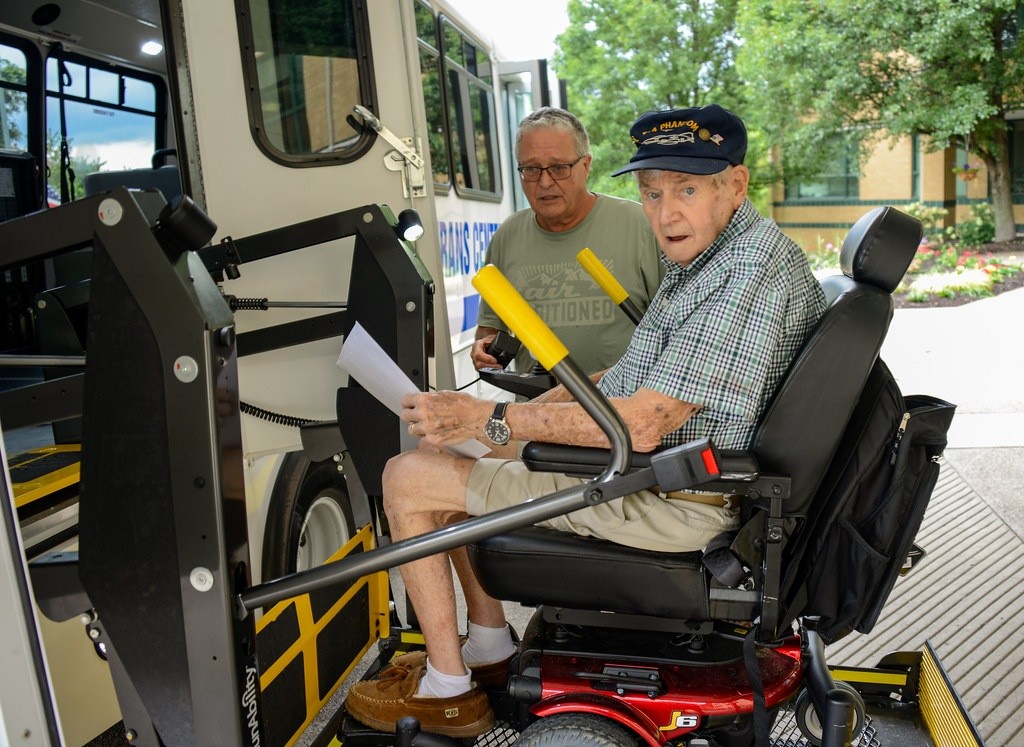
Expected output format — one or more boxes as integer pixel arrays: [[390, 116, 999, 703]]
[[390, 636, 523, 689], [346, 666, 496, 737]]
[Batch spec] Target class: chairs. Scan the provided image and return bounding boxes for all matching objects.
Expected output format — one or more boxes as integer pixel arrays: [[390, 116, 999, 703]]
[[337, 205, 925, 747], [0, 148, 96, 358]]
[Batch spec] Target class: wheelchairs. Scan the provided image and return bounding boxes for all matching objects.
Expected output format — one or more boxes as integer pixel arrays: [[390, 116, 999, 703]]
[[346, 205, 952, 741]]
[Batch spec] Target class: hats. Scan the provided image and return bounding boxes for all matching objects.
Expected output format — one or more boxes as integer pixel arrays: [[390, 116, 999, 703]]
[[609, 104, 748, 177]]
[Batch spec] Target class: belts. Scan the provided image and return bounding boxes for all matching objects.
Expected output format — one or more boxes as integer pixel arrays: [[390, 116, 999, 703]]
[[649, 482, 743, 508]]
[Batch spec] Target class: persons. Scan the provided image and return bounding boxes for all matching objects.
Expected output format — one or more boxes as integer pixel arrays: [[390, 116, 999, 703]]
[[471, 108, 667, 404], [343, 105, 832, 741]]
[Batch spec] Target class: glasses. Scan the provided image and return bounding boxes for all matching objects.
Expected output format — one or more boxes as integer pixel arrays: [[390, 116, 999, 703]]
[[518, 158, 583, 182]]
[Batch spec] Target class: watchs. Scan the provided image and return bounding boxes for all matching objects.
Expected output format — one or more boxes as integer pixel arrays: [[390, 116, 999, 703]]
[[484, 399, 513, 446]]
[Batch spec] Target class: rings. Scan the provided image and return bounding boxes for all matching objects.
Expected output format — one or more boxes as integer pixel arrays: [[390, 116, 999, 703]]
[[411, 423, 417, 436]]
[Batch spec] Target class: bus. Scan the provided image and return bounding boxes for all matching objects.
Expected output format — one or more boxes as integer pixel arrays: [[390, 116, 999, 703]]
[[0, 2, 556, 747]]
